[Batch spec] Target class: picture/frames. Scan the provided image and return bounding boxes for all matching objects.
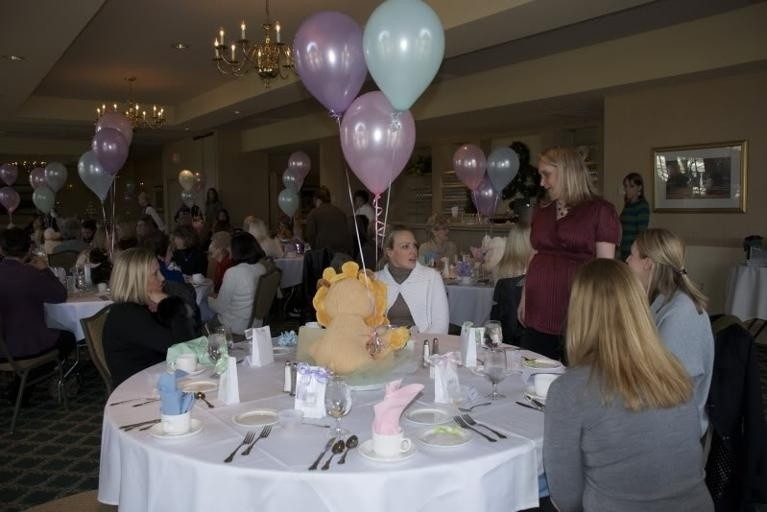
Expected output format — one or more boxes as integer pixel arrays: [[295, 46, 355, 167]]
[[646, 138, 752, 215]]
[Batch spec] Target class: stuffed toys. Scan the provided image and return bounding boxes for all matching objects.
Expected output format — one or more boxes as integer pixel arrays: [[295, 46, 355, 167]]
[[307, 262, 410, 375]]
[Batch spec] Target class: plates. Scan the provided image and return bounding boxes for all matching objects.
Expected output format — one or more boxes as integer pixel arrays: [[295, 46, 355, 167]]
[[417, 428, 475, 446], [522, 358, 562, 370], [524, 392, 547, 399], [405, 407, 452, 425], [232, 407, 280, 426], [270, 345, 293, 355], [181, 379, 219, 392], [151, 415, 204, 439], [444, 349, 512, 377], [360, 439, 417, 460], [167, 363, 205, 376]]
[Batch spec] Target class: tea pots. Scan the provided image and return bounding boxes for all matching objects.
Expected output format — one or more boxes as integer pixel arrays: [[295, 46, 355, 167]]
[[293, 239, 304, 256]]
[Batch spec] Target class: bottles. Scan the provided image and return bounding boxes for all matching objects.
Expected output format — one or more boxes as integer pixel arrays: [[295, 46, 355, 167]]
[[51, 263, 92, 296], [422, 340, 430, 367], [282, 360, 291, 393], [288, 363, 297, 395], [431, 338, 439, 355]]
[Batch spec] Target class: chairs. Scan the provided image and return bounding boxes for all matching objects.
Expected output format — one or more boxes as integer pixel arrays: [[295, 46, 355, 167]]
[[79, 304, 119, 399], [2, 332, 70, 437], [47, 250, 82, 270], [247, 268, 283, 330], [700, 313, 765, 510]]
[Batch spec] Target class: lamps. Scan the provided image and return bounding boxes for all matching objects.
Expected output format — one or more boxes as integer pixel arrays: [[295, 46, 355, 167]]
[[96, 75, 176, 143], [212, 0, 299, 94]]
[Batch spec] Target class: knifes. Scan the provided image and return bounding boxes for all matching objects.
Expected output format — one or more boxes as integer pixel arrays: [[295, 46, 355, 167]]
[[515, 395, 545, 411], [119, 419, 160, 434], [309, 436, 334, 470]]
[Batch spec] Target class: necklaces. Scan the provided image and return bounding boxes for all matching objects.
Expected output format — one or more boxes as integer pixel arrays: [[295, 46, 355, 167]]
[[555, 203, 568, 217]]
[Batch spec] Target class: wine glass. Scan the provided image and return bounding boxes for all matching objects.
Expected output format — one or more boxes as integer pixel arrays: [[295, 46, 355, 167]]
[[481, 320, 502, 351], [207, 325, 232, 378], [325, 382, 351, 438], [483, 347, 507, 399]]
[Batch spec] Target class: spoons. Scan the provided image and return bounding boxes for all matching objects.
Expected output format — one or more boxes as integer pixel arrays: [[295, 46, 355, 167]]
[[240, 425, 272, 455], [321, 439, 343, 471], [457, 402, 490, 413], [336, 434, 358, 464]]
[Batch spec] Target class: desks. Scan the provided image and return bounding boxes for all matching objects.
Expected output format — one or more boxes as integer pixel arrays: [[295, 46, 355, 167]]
[[725, 254, 765, 323], [268, 254, 305, 315], [96, 323, 569, 510], [438, 265, 497, 334], [41, 273, 219, 385]]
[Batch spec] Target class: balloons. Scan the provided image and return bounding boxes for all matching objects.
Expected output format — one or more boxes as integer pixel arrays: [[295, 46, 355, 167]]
[[29, 162, 68, 213], [277, 150, 311, 219], [2, 163, 20, 214], [362, 2, 447, 113], [180, 170, 205, 206], [340, 91, 415, 195], [77, 112, 133, 201], [452, 143, 519, 218], [291, 12, 367, 117]]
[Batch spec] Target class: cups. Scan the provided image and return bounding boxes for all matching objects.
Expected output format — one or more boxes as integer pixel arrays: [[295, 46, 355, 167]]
[[533, 373, 561, 395], [305, 321, 320, 329], [160, 410, 191, 435], [95, 283, 108, 294], [191, 273, 202, 284], [278, 410, 304, 447], [172, 353, 195, 372], [429, 254, 489, 285], [371, 426, 410, 455]]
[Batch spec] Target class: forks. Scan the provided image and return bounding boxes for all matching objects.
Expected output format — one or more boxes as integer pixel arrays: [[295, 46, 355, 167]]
[[197, 391, 213, 408], [451, 416, 497, 442], [461, 413, 506, 438], [225, 430, 254, 463]]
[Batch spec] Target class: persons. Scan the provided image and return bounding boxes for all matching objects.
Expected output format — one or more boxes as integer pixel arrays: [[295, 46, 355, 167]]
[[200, 231, 266, 335], [518, 145, 618, 362], [352, 191, 376, 270], [0, 227, 78, 397], [618, 171, 650, 262], [627, 227, 715, 451], [543, 259, 717, 512], [101, 247, 201, 388], [491, 222, 535, 344], [371, 226, 450, 334], [1, 208, 138, 284], [418, 213, 458, 267], [136, 189, 304, 294], [303, 188, 348, 277]]
